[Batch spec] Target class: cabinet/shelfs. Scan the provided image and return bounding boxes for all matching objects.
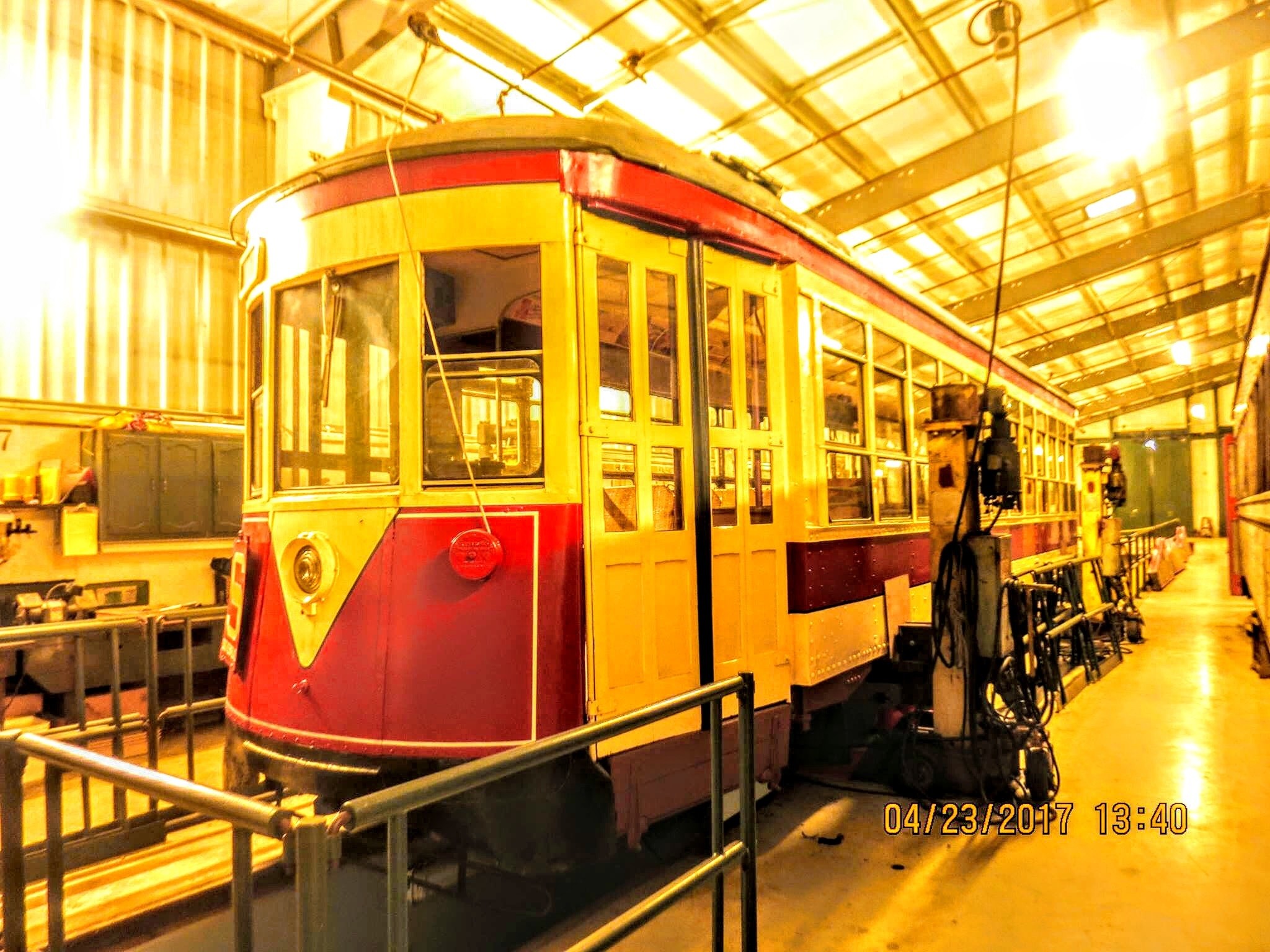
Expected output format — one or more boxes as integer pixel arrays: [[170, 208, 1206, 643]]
[[80, 429, 243, 542]]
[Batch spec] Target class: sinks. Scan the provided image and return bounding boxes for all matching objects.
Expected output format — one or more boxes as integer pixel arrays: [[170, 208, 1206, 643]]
[[28, 601, 97, 619]]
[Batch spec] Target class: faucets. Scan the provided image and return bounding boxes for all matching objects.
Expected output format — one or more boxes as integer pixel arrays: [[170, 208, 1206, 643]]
[[45, 583, 72, 601]]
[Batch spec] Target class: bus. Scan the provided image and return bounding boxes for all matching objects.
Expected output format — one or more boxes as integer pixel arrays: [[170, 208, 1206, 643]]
[[213, 112, 1132, 902], [1221, 229, 1270, 678]]
